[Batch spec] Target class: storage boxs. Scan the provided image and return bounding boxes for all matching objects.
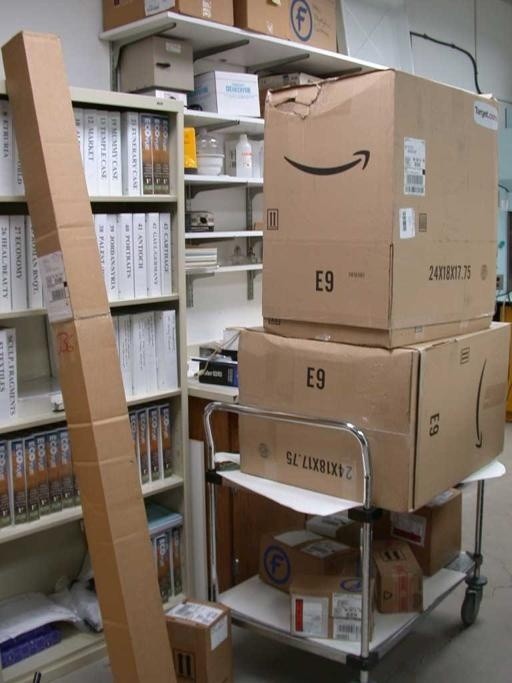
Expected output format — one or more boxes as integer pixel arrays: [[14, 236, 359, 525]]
[[102, 0, 339, 51]]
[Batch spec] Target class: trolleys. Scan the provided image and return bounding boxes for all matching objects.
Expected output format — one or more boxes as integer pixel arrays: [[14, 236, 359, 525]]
[[199, 399, 508, 681]]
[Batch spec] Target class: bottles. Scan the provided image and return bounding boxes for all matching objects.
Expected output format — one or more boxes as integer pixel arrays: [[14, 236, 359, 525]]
[[236, 132, 252, 177], [197, 127, 227, 176]]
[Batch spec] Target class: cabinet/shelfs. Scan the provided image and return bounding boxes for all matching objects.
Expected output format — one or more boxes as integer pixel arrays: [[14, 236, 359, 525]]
[[1, 80, 187, 681], [100, 12, 391, 306]]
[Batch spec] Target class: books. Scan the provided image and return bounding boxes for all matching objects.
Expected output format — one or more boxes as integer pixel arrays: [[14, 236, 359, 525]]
[[0, 310, 178, 426], [188, 245, 216, 274], [80, 500, 182, 604], [1, 212, 173, 314], [1, 402, 172, 528], [2, 101, 170, 196]]
